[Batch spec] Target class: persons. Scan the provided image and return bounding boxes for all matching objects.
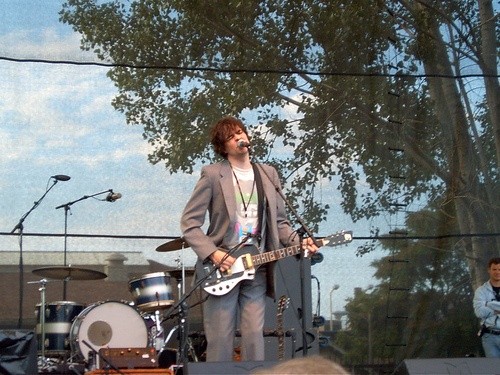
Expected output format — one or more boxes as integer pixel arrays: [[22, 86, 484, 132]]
[[473, 258, 500, 357], [179, 116, 319, 362]]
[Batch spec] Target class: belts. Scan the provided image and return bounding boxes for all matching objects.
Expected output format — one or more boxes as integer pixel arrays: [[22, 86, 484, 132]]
[[483, 329, 500, 335]]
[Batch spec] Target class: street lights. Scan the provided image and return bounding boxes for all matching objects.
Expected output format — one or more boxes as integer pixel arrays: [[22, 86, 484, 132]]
[[329, 283, 339, 331]]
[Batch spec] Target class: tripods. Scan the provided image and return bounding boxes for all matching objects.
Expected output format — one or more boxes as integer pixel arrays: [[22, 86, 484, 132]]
[[158, 278, 199, 363]]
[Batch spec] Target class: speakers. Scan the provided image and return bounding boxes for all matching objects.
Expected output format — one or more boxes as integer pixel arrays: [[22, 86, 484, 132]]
[[175, 361, 280, 375], [390, 358, 500, 375]]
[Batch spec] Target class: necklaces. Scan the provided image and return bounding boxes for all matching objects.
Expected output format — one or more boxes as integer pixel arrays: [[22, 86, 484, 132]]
[[228, 162, 256, 218]]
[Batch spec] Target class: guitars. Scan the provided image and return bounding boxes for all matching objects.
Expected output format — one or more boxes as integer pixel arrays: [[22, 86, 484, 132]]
[[274, 294, 291, 362], [194, 229, 353, 297]]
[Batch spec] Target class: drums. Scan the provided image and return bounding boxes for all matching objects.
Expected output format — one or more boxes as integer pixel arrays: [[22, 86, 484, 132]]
[[127, 271, 176, 313], [34, 300, 87, 359], [69, 298, 149, 370]]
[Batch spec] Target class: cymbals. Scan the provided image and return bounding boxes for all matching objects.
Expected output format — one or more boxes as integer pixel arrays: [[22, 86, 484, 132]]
[[31, 266, 108, 281], [168, 270, 196, 280], [155, 238, 191, 252]]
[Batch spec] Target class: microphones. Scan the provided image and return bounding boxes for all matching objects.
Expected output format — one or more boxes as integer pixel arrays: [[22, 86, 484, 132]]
[[238, 139, 251, 148], [257, 231, 261, 240], [52, 175, 70, 181], [107, 192, 121, 202]]
